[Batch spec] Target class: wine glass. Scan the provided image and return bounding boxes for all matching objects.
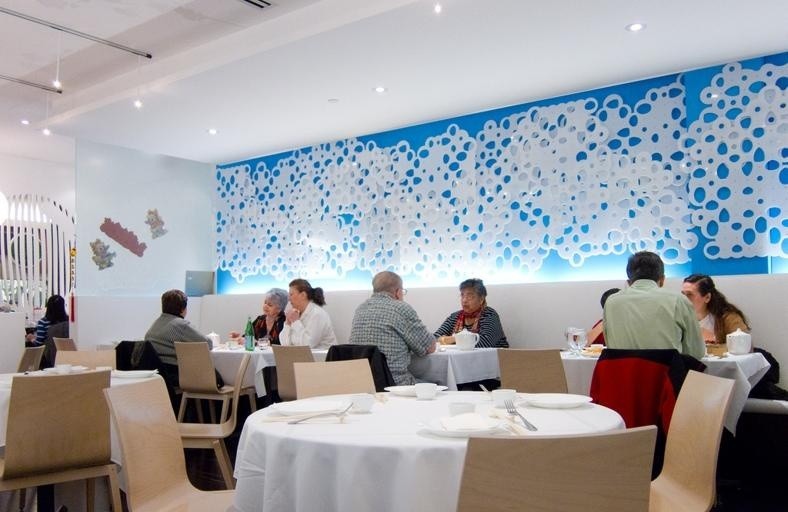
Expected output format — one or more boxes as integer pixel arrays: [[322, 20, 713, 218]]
[[568, 329, 586, 358]]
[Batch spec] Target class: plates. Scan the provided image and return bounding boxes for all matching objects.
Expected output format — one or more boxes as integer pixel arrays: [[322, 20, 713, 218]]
[[276, 385, 593, 436], [24, 366, 159, 378]]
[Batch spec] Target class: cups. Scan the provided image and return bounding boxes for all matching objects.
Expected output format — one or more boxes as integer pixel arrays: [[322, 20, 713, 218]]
[[227, 339, 238, 351], [258, 338, 270, 351], [435, 343, 440, 352], [350, 382, 516, 417], [591, 344, 603, 349]]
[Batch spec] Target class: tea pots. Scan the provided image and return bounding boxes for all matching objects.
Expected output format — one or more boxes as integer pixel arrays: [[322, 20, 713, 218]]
[[453, 328, 479, 351], [726, 328, 752, 354], [206, 332, 220, 349]]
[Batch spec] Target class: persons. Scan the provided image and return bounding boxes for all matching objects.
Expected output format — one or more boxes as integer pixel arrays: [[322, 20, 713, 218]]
[[278, 279, 338, 349], [351, 271, 436, 386], [681, 274, 751, 345], [584, 288, 621, 346], [228, 287, 289, 345], [433, 278, 509, 391], [603, 250, 706, 360], [26, 295, 69, 370], [145, 290, 213, 389]]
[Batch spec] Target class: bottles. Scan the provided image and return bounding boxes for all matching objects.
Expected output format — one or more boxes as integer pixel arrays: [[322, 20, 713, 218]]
[[244, 317, 255, 351]]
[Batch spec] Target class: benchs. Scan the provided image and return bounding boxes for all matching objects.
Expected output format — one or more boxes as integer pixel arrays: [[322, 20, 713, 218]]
[[202, 272, 788, 414]]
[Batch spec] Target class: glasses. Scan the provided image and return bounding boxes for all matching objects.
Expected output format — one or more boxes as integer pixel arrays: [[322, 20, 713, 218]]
[[400, 287, 407, 296]]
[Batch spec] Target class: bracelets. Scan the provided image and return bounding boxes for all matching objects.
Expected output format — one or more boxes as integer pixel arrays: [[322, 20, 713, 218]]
[[441, 336, 446, 346]]
